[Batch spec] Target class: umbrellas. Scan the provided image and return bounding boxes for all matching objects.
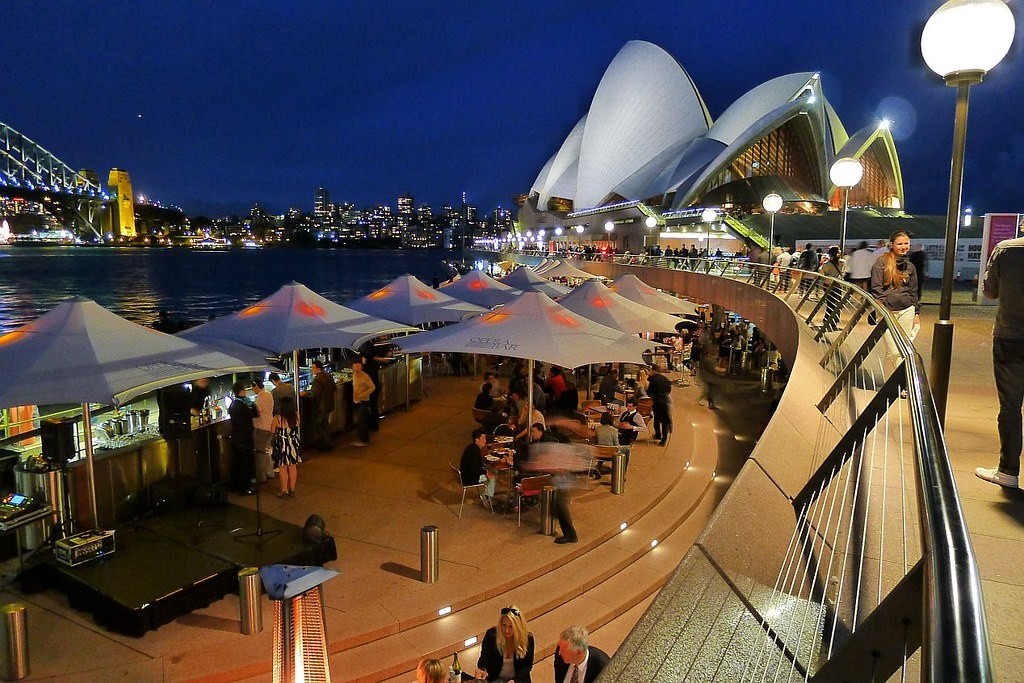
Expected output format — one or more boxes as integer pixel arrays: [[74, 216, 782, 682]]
[[344, 258, 703, 442], [0, 296, 287, 529], [174, 278, 421, 444]]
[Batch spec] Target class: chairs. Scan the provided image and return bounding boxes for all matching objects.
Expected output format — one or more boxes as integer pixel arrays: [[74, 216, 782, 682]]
[[448, 358, 653, 528]]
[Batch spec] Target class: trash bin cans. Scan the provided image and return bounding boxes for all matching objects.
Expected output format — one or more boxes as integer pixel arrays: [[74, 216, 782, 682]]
[[740, 351, 749, 378], [761, 367, 774, 397]]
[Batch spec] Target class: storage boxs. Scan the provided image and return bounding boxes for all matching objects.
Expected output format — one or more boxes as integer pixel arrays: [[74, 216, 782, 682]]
[[55, 531, 115, 567]]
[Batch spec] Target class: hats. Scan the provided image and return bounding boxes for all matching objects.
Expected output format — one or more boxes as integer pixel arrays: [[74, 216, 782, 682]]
[[651, 364, 661, 372]]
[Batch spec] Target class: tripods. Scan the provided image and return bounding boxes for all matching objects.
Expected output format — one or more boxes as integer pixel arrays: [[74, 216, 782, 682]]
[[222, 438, 281, 550], [177, 424, 225, 544], [24, 462, 85, 563]]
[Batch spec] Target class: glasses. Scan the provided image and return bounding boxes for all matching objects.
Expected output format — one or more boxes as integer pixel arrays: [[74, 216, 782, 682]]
[[501, 608, 521, 620]]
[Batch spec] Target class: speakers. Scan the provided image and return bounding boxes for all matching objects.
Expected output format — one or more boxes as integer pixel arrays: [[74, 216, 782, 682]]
[[40, 417, 76, 462]]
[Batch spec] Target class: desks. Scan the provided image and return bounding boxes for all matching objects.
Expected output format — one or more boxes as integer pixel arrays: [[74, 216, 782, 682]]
[[581, 424, 621, 444], [589, 406, 629, 418]]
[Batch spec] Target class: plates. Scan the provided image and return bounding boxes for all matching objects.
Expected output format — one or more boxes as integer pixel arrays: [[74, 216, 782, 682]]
[[487, 456, 500, 462]]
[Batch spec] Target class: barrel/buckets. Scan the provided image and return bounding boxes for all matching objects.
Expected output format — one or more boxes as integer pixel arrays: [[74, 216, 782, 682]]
[[104, 409, 151, 437], [13, 462, 70, 551]]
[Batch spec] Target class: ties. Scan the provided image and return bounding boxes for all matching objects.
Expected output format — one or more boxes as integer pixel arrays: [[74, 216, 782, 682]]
[[624, 412, 630, 418], [570, 665, 578, 683]]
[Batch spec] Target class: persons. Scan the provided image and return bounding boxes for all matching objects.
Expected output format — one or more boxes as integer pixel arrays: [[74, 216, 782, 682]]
[[475, 604, 535, 683], [519, 239, 889, 293], [308, 360, 337, 451], [270, 396, 301, 498], [820, 245, 845, 332], [416, 658, 484, 683], [251, 377, 275, 482], [228, 382, 261, 497], [870, 230, 920, 384], [908, 243, 927, 316], [350, 361, 375, 446], [189, 378, 211, 416], [974, 216, 1024, 489], [360, 354, 381, 432], [718, 322, 781, 377], [459, 360, 673, 544], [268, 372, 295, 418], [553, 625, 610, 683]]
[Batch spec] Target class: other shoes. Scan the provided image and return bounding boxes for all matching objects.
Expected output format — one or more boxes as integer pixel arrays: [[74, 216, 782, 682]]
[[278, 491, 288, 498], [481, 494, 490, 509], [554, 534, 578, 544], [288, 490, 294, 497], [351, 441, 367, 447], [652, 434, 656, 439], [239, 489, 255, 496], [657, 441, 665, 447], [252, 478, 257, 482]]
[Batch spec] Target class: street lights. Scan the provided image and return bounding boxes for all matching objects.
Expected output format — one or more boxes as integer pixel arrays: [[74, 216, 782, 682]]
[[920, 1, 1017, 439], [576, 224, 584, 253], [762, 194, 783, 291], [829, 157, 865, 263], [702, 208, 717, 271], [604, 222, 615, 261], [645, 216, 659, 265], [555, 228, 562, 251]]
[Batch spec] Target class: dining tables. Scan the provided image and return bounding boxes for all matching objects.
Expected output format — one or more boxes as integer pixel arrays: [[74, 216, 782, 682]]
[[488, 463, 515, 516]]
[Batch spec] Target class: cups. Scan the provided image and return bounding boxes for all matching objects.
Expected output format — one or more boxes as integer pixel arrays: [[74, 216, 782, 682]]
[[478, 668, 486, 680]]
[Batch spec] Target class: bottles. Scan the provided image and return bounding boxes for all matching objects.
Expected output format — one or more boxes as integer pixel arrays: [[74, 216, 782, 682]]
[[607, 403, 615, 411], [452, 651, 462, 683], [198, 395, 222, 426]]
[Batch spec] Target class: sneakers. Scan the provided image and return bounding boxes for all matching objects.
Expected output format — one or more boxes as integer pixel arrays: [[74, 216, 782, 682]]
[[976, 466, 1018, 489]]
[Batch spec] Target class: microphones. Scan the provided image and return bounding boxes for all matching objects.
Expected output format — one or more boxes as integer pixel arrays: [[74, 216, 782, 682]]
[[169, 420, 186, 424], [216, 434, 232, 438]]
